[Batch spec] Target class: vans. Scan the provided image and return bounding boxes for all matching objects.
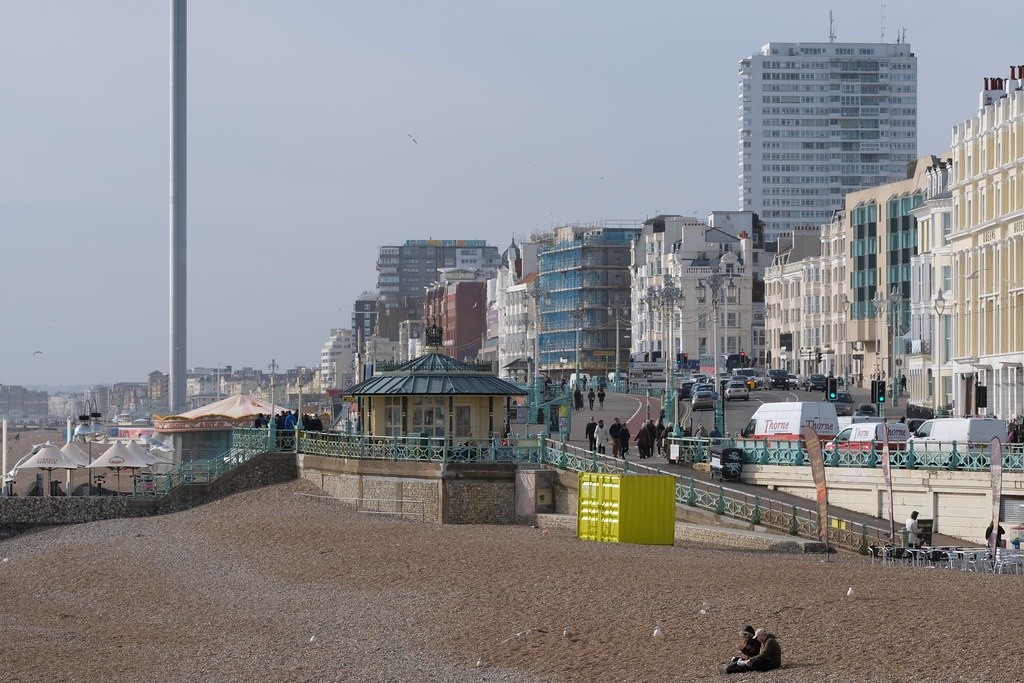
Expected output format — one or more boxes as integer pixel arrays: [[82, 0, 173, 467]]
[[824, 422, 911, 466], [823, 392, 856, 415], [904, 418, 1010, 466], [741, 400, 839, 452]]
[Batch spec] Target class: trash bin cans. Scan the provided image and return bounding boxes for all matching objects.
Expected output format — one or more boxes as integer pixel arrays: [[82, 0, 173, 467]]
[[708, 446, 746, 482]]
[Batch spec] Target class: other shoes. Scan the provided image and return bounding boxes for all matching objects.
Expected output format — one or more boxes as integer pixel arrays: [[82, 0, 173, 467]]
[[717, 663, 725, 675]]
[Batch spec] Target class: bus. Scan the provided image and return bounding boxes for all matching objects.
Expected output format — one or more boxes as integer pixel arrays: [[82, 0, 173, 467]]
[[700, 353, 750, 377]]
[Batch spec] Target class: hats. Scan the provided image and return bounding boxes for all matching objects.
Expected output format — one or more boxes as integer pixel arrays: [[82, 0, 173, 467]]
[[753, 629, 766, 639]]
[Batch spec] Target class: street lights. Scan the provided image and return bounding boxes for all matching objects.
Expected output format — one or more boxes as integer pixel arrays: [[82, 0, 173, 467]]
[[841, 294, 850, 391], [932, 288, 953, 418], [519, 270, 738, 446], [295, 371, 307, 430], [72, 398, 106, 486], [267, 359, 280, 417], [872, 290, 887, 380], [890, 284, 905, 407]]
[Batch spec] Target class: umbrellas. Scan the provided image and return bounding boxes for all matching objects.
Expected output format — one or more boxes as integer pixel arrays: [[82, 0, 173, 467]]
[[15, 440, 175, 497]]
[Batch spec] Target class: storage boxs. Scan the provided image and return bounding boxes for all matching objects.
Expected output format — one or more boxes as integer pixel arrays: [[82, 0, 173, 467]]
[[575, 472, 677, 546]]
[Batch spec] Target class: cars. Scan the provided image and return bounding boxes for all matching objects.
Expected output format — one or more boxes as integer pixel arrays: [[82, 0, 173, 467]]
[[854, 403, 877, 416], [676, 368, 801, 412], [903, 419, 927, 437], [805, 374, 827, 393]]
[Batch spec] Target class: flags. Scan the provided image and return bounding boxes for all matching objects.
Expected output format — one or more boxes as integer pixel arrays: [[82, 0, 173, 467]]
[[988, 437, 1002, 557], [805, 426, 828, 549], [882, 419, 893, 540]]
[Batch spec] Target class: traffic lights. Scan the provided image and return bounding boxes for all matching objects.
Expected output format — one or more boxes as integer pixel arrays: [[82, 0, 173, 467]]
[[677, 353, 680, 365], [877, 380, 886, 403], [818, 352, 823, 363], [683, 353, 689, 364], [828, 378, 838, 400], [740, 351, 746, 364]]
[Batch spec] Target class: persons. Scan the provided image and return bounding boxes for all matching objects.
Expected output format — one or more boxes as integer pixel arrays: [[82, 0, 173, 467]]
[[255, 409, 324, 451], [898, 417, 916, 432], [524, 372, 587, 391], [827, 371, 834, 378], [1007, 427, 1022, 443], [716, 626, 781, 674], [993, 416, 998, 419], [986, 518, 1005, 568], [906, 511, 924, 560], [344, 409, 358, 434], [573, 387, 605, 411], [465, 433, 473, 446], [585, 416, 722, 465], [901, 374, 906, 392]]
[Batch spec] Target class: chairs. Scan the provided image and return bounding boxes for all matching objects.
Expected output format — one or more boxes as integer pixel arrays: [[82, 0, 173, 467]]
[[868, 544, 1024, 576]]
